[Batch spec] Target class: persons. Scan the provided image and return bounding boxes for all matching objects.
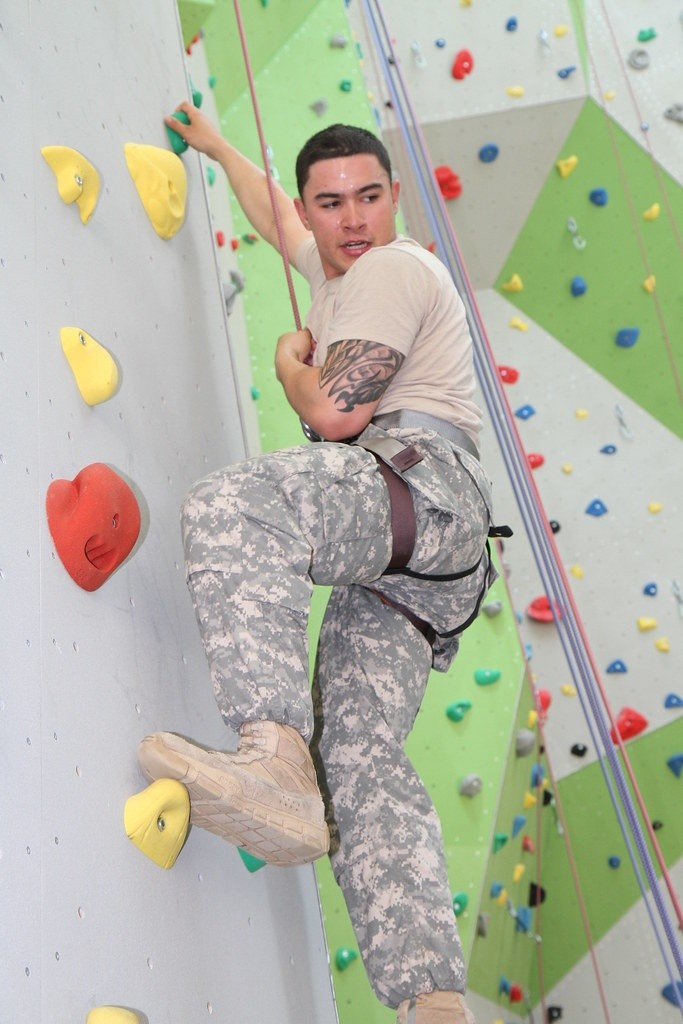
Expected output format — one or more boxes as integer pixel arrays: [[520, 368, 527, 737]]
[[138, 102, 498, 1024]]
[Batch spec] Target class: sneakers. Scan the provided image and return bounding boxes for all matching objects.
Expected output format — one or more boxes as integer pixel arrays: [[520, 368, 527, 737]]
[[393, 992, 476, 1024], [140, 719, 332, 866]]
